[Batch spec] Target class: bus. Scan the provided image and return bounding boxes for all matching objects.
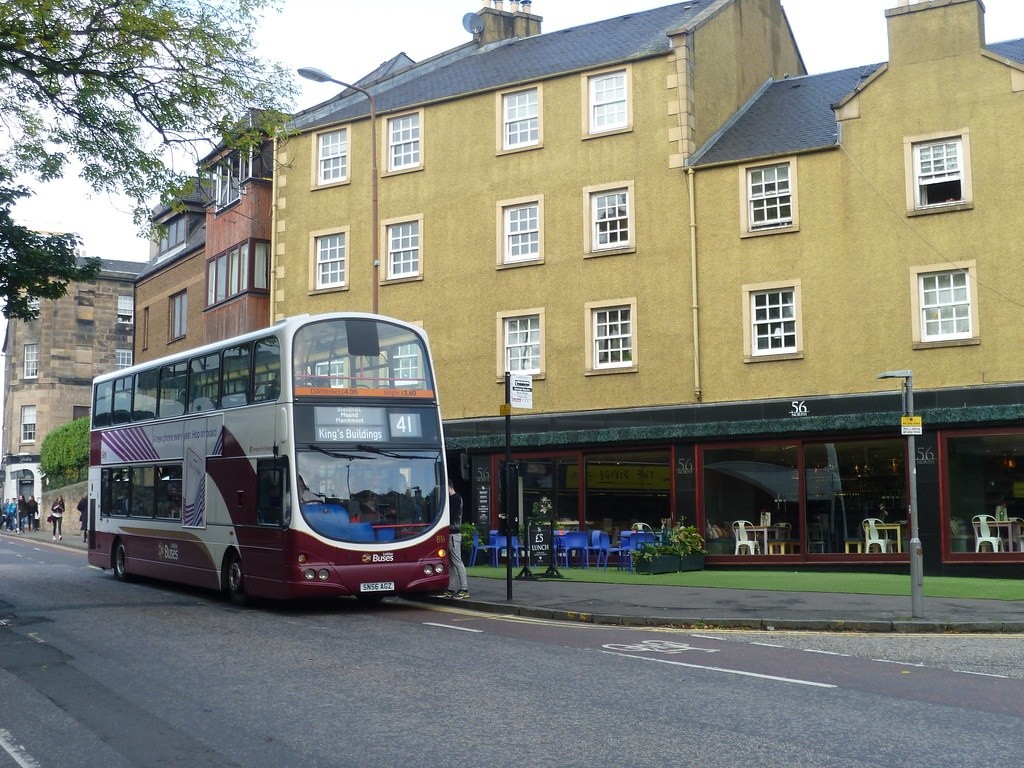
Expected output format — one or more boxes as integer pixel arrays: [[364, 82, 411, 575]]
[[87, 311, 451, 608]]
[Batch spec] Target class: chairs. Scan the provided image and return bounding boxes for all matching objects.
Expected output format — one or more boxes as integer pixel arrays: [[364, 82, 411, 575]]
[[768, 523, 792, 554], [1001, 517, 1024, 553], [807, 523, 826, 554], [552, 523, 669, 574], [469, 530, 525, 566], [972, 514, 1005, 552], [862, 518, 893, 553], [731, 520, 761, 555]]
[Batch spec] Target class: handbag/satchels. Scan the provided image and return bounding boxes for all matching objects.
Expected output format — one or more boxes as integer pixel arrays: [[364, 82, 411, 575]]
[[47, 515, 53, 524], [79, 516, 82, 522]]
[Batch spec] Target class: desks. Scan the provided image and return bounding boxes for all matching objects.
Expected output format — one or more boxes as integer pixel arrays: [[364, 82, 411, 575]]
[[735, 525, 790, 556], [973, 521, 1024, 552], [865, 523, 908, 553], [951, 534, 974, 552]]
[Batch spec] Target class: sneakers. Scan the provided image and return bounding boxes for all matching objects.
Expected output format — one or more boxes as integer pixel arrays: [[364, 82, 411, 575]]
[[435, 588, 455, 598], [451, 589, 470, 600]]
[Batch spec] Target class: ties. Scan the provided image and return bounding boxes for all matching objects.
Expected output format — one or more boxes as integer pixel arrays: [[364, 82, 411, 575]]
[[302, 497, 305, 502]]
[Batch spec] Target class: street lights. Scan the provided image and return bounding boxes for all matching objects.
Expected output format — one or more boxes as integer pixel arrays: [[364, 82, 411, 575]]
[[298, 68, 380, 388], [877, 370, 924, 617]]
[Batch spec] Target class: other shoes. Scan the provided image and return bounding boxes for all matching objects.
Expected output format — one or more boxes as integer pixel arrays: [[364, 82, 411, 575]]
[[53, 536, 56, 540], [59, 535, 62, 540]]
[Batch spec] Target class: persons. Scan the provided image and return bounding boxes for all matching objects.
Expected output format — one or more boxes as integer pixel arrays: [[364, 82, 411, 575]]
[[101, 468, 182, 519], [0, 495, 39, 534], [52, 495, 65, 541], [77, 492, 88, 543], [435, 479, 469, 601], [283, 475, 322, 506], [359, 473, 415, 531]]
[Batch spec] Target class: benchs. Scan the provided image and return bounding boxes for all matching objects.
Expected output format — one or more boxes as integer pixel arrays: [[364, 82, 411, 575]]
[[768, 539, 800, 555], [844, 538, 865, 554]]
